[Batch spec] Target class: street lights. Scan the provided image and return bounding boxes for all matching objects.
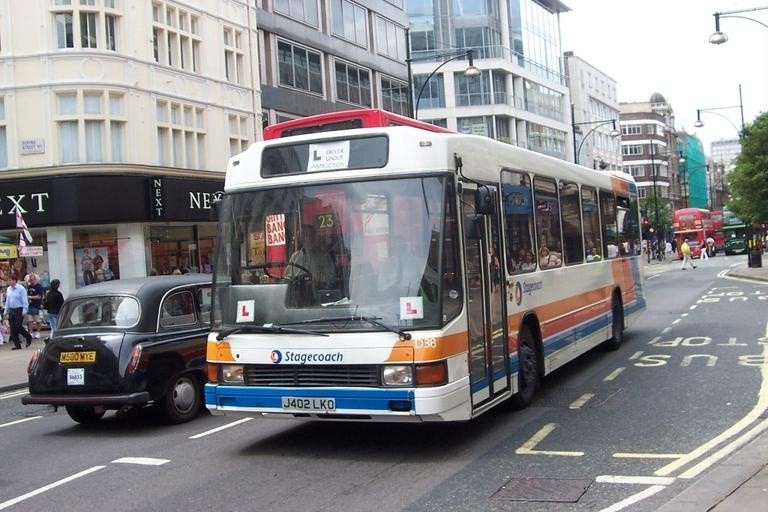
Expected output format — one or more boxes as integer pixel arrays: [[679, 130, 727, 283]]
[[405, 25, 481, 121], [569, 101, 620, 164], [649, 138, 687, 261], [708, 4, 768, 46], [680, 160, 710, 208], [695, 83, 745, 147]]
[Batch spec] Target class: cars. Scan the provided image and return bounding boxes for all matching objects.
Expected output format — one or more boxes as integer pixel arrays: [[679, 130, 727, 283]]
[[21, 273, 254, 423]]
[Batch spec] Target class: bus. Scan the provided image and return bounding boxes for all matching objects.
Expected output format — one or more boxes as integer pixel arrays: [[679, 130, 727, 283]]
[[262, 109, 463, 284], [203, 107, 647, 425], [723, 207, 752, 256], [710, 209, 727, 253], [673, 208, 716, 259]]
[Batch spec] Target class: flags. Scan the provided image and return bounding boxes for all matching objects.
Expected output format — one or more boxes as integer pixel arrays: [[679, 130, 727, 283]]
[[15, 206, 34, 251]]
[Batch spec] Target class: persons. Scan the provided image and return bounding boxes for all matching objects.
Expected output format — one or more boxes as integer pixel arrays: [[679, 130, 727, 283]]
[[248, 270, 261, 283], [671, 237, 717, 271], [2, 273, 32, 351], [284, 223, 337, 291], [96, 301, 117, 326], [585, 234, 596, 254], [8, 255, 27, 283], [149, 266, 190, 276], [377, 234, 439, 294], [91, 248, 104, 280], [82, 303, 99, 321], [46, 279, 66, 341], [466, 245, 563, 341], [25, 273, 43, 340], [81, 246, 93, 285], [590, 247, 601, 260]]
